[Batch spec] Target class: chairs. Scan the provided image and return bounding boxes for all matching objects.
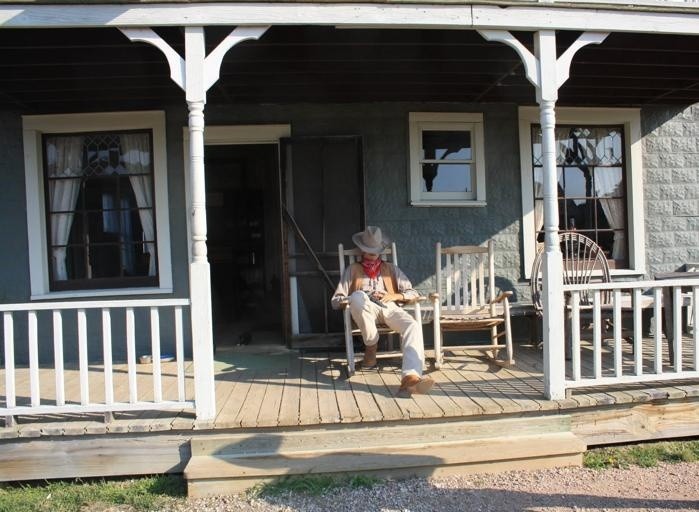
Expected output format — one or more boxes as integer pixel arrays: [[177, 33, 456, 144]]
[[428, 238, 519, 370], [532, 232, 633, 361], [336, 240, 429, 376]]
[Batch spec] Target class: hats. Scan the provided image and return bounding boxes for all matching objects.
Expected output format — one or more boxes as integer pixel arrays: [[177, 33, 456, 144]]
[[352, 226, 391, 254]]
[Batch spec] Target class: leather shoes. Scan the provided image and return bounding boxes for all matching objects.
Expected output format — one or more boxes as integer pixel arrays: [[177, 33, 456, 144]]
[[400, 376, 434, 398], [365, 345, 377, 366]]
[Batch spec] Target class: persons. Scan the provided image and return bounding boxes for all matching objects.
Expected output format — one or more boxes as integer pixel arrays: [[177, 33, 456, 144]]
[[330, 225, 436, 398]]
